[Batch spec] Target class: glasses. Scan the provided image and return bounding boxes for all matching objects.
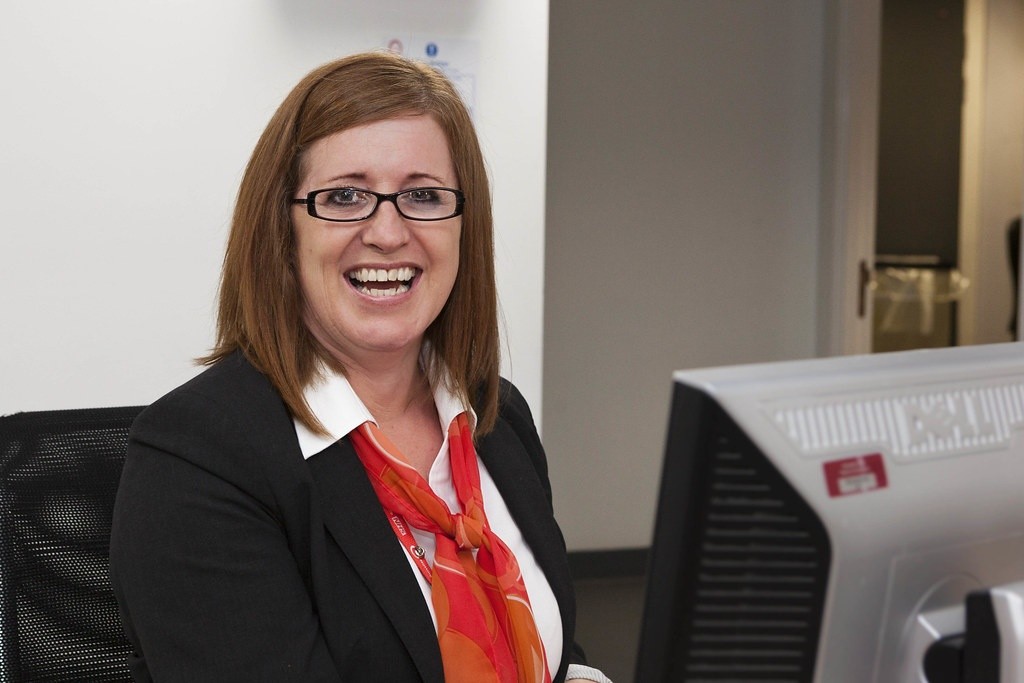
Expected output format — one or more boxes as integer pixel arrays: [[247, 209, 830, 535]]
[[291, 186, 466, 222]]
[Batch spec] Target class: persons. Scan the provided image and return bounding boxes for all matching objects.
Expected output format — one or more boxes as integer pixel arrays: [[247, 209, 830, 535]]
[[109, 53, 612, 683], [1005, 217, 1023, 342]]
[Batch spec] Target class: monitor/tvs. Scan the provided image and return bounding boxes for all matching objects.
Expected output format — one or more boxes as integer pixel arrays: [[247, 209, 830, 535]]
[[631, 341, 1024, 683]]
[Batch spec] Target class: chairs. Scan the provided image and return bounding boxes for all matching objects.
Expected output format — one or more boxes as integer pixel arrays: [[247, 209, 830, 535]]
[[0, 406, 144, 683]]
